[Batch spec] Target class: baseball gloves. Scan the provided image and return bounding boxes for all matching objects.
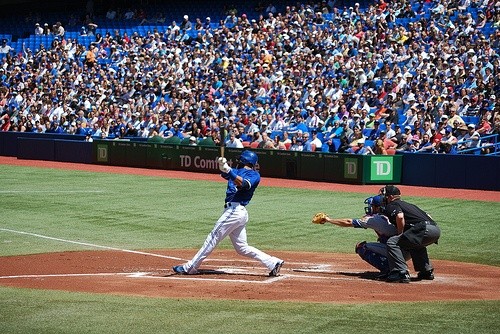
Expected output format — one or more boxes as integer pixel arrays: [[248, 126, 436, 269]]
[[312, 212, 328, 224]]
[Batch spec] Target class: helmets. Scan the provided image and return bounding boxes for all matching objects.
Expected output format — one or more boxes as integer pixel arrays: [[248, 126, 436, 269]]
[[383, 186, 402, 196], [239, 149, 259, 166], [370, 194, 385, 206]]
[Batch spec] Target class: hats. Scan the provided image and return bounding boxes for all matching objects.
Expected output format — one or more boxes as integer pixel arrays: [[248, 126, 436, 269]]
[[0, 1, 500, 155]]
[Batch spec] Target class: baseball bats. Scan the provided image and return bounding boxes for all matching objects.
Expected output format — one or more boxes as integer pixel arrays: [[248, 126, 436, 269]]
[[219, 126, 225, 158]]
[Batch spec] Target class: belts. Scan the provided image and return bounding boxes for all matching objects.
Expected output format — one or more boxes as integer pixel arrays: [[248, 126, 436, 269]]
[[224, 202, 244, 209], [425, 220, 437, 226]]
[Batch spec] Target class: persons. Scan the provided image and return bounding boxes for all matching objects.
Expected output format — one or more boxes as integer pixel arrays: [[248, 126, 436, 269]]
[[0, 0, 500, 158], [322, 195, 412, 279], [379, 185, 441, 283], [172, 150, 285, 276]]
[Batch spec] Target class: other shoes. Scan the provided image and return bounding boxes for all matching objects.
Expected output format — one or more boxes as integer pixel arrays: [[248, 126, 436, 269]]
[[374, 272, 388, 281], [417, 268, 434, 280], [385, 270, 411, 284], [172, 265, 186, 275], [268, 259, 285, 277]]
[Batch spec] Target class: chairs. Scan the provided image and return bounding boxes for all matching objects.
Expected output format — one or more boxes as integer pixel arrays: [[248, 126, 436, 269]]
[[0, 0, 500, 156]]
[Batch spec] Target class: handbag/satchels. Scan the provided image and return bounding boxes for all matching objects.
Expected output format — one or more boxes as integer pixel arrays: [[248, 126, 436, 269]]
[[398, 225, 426, 247]]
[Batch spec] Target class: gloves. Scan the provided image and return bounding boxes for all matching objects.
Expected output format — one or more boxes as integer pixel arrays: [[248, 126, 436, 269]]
[[220, 163, 232, 173], [218, 157, 227, 166]]
